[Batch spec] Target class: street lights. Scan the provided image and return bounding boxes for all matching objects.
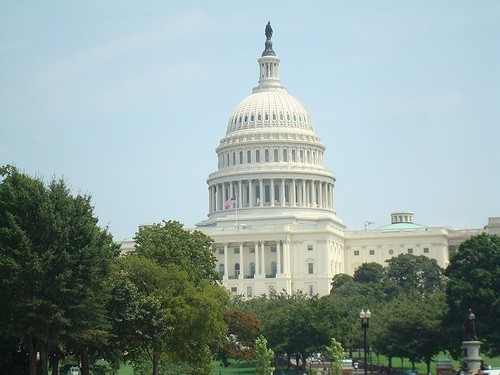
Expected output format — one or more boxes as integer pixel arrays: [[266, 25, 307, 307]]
[[359, 308, 372, 375]]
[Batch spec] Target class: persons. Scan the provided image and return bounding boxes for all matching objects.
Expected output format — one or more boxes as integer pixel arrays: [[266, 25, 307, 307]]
[[462, 359, 470, 375], [265, 22, 273, 40], [318, 357, 321, 364], [378, 363, 385, 375], [354, 361, 359, 372], [322, 367, 328, 375], [464, 309, 477, 340], [311, 354, 315, 362]]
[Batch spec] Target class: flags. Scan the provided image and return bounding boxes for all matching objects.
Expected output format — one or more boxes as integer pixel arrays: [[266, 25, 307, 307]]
[[224, 196, 236, 206]]
